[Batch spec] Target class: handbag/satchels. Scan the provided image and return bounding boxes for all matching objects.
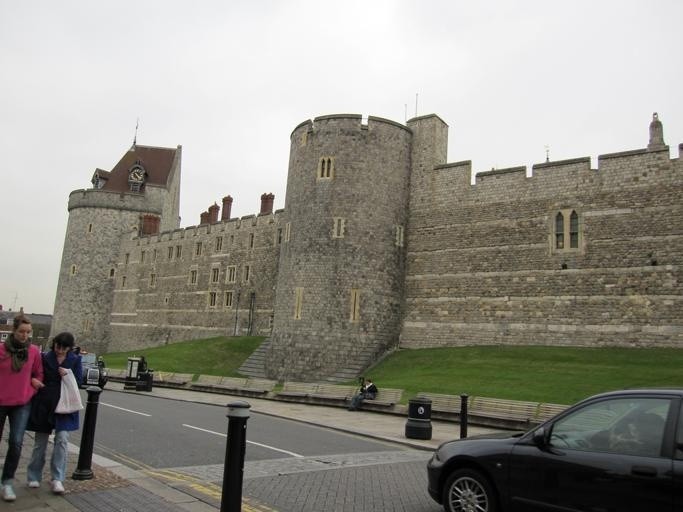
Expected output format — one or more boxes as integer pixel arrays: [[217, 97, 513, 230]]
[[365, 393, 375, 400], [54, 368, 83, 414]]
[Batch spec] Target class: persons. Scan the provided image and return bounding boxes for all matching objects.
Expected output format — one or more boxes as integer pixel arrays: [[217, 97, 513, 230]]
[[25, 330, 84, 495], [0, 314, 44, 503]]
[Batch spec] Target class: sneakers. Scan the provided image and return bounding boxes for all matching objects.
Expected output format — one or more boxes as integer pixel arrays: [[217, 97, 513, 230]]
[[51, 479, 65, 493], [2, 484, 16, 502], [28, 480, 40, 488]]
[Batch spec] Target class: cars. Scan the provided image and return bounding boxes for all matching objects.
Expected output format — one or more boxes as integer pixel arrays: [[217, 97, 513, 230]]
[[78, 351, 108, 391], [423, 389, 682, 512]]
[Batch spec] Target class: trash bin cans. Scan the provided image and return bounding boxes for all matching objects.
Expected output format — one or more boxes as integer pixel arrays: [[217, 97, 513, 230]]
[[135, 372, 153, 392], [405, 397, 433, 440]]
